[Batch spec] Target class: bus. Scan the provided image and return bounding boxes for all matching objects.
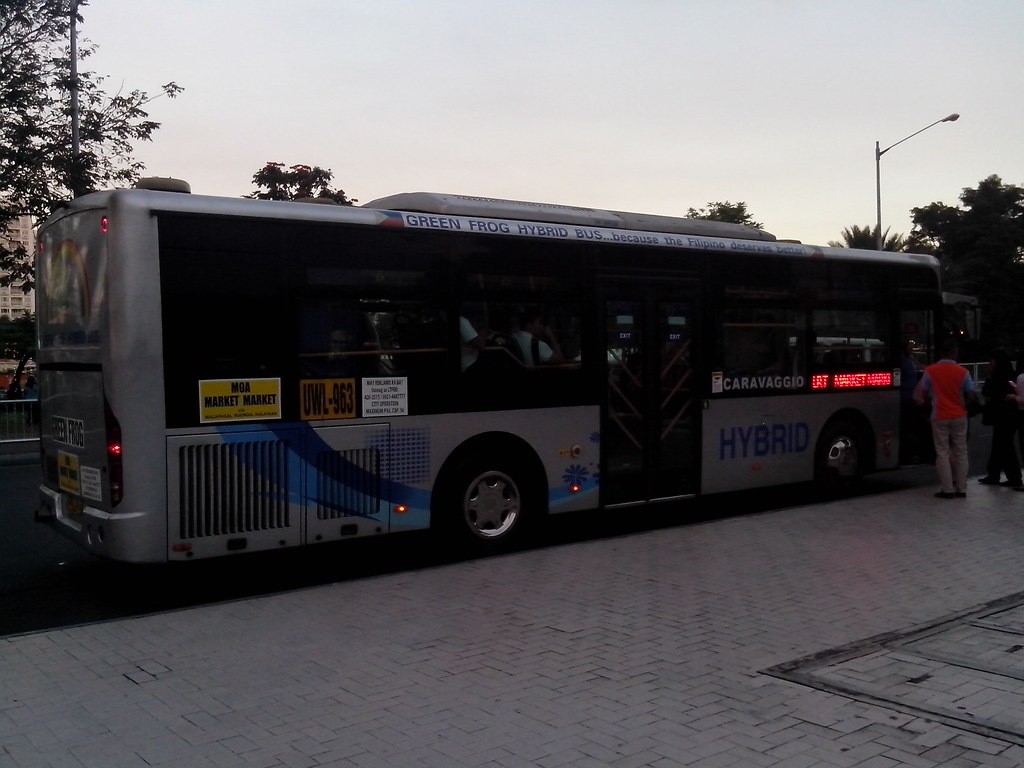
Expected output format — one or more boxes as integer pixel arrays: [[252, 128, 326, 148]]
[[33, 175, 983, 568]]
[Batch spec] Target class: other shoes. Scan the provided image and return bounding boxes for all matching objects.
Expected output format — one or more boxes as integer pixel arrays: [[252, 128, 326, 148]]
[[978, 476, 1024, 489], [934, 489, 966, 499]]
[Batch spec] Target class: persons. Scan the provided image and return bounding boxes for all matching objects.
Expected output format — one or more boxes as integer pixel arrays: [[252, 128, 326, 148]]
[[981, 347, 1024, 492], [913, 343, 976, 500], [321, 312, 917, 469]]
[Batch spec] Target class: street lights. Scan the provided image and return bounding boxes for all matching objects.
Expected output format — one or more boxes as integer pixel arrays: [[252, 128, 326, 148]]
[[875, 113, 961, 251]]
